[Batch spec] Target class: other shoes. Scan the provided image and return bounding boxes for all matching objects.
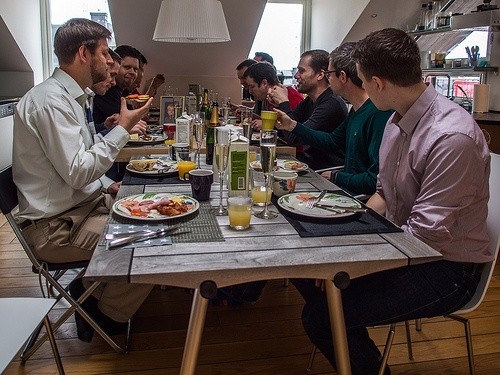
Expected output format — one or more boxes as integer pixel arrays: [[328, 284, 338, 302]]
[[68, 277, 98, 343], [228, 280, 267, 305], [94, 312, 131, 352]]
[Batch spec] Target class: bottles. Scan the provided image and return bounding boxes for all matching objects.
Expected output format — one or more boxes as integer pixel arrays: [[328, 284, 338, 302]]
[[417, 0, 451, 30], [205, 100, 221, 166], [197, 88, 211, 135]]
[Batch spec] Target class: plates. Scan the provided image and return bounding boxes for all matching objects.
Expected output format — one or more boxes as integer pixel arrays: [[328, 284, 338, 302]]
[[277, 193, 361, 218], [250, 159, 309, 173], [251, 133, 274, 141], [128, 107, 168, 144], [112, 193, 200, 220], [126, 160, 179, 174]]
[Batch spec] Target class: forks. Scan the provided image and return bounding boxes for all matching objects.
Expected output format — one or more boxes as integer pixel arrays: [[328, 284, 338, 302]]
[[306, 189, 327, 209]]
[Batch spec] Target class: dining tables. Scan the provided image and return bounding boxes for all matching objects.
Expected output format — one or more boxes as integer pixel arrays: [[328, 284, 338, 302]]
[[82, 104, 444, 375]]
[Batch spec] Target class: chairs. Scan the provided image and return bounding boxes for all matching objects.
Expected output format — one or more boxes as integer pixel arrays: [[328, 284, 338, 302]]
[[307, 153, 500, 375], [0, 162, 138, 363]]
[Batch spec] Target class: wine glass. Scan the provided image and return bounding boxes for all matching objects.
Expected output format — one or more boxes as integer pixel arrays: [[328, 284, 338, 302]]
[[209, 127, 232, 216], [254, 130, 278, 219], [190, 112, 205, 169]]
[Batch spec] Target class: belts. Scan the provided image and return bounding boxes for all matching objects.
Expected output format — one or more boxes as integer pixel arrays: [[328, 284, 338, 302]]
[[18, 216, 43, 230]]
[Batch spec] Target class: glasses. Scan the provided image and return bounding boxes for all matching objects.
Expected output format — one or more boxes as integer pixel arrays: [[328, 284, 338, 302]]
[[322, 69, 350, 80]]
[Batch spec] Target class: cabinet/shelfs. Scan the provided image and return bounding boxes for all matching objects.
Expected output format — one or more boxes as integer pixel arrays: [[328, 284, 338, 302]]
[[404, 24, 500, 155]]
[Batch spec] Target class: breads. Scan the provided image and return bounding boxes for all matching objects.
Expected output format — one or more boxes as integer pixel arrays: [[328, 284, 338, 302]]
[[124, 94, 149, 106], [131, 160, 155, 170]]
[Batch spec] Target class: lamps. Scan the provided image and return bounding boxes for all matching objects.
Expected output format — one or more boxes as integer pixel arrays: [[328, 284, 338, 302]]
[[151, 0, 231, 44]]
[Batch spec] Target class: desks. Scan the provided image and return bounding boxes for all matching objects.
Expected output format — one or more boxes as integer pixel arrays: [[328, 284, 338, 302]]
[[0, 297, 65, 375]]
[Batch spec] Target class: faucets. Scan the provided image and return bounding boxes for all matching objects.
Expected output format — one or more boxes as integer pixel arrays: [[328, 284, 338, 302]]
[[424, 73, 450, 98]]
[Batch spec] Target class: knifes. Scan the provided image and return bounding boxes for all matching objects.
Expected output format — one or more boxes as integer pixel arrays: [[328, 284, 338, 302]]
[[315, 203, 368, 213], [109, 223, 191, 248]]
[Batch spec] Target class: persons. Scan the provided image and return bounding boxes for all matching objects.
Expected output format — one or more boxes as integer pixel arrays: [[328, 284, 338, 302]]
[[92, 45, 165, 182], [212, 43, 396, 306], [301, 28, 491, 375], [13, 18, 154, 343]]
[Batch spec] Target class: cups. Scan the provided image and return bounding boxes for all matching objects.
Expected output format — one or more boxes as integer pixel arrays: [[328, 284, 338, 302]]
[[250, 161, 273, 208], [270, 171, 298, 198], [243, 124, 254, 145], [261, 110, 278, 130], [175, 149, 197, 181], [183, 169, 214, 202], [221, 97, 253, 125], [171, 143, 190, 160], [419, 51, 480, 69], [227, 196, 252, 231], [163, 131, 177, 148], [163, 123, 176, 131]]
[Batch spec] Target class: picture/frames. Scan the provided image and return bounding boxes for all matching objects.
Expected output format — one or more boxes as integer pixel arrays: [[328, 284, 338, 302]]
[[159, 95, 186, 126]]
